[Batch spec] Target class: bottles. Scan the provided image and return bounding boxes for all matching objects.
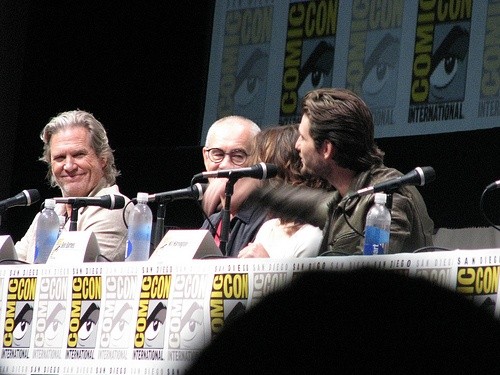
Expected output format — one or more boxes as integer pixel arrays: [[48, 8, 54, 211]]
[[363, 193, 392, 255], [125, 192, 153, 261], [34, 199, 60, 264]]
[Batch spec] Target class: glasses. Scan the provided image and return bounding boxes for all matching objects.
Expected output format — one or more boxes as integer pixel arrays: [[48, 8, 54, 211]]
[[205, 148, 251, 166]]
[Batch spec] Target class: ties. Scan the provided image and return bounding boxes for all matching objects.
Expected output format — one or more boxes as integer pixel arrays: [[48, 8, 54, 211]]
[[214, 219, 222, 248]]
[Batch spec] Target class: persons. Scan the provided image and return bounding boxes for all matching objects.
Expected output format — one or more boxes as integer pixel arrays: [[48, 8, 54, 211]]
[[200, 116, 272, 258], [237, 123, 337, 258], [181, 264, 500, 375], [201, 88, 435, 257], [13, 110, 135, 264]]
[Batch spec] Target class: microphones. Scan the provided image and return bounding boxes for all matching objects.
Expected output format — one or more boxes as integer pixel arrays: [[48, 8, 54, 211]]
[[0, 189, 41, 213], [53, 194, 125, 210], [132, 183, 210, 203], [194, 162, 277, 180], [348, 165, 437, 200]]
